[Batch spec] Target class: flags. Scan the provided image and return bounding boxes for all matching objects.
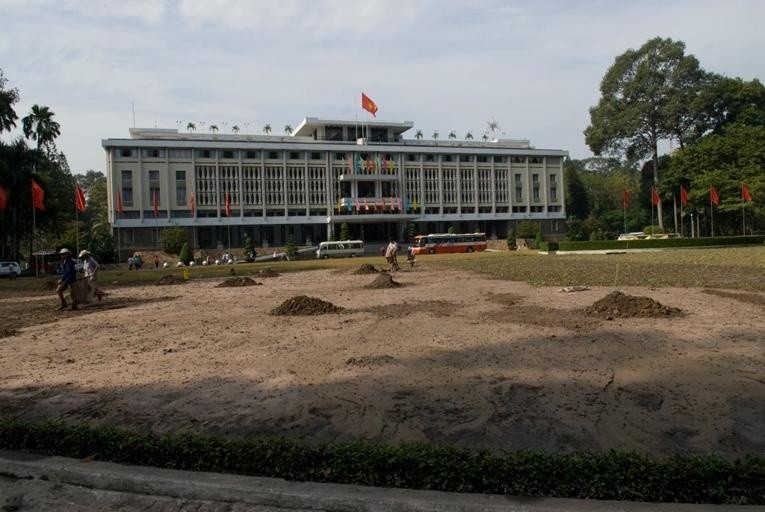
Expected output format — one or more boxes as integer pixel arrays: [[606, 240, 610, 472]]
[[226, 193, 232, 216], [116, 192, 124, 215], [75, 182, 86, 212], [362, 93, 378, 117], [0, 185, 6, 209], [624, 184, 751, 206], [191, 193, 195, 216], [32, 179, 46, 211], [153, 193, 158, 214]]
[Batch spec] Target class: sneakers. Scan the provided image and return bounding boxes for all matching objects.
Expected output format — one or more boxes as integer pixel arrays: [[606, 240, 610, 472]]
[[67, 305, 79, 311], [58, 305, 68, 311]]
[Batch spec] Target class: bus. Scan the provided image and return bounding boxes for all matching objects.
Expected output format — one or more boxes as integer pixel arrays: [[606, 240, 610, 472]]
[[29, 250, 57, 274], [409, 232, 487, 256]]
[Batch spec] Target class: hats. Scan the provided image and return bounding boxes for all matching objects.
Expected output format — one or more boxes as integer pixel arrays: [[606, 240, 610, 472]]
[[78, 249, 91, 258], [58, 248, 72, 254]]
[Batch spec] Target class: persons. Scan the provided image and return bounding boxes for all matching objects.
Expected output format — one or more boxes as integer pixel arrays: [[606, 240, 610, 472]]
[[155, 256, 159, 268], [57, 249, 78, 311], [223, 252, 229, 263], [134, 255, 141, 270], [128, 257, 133, 270], [386, 236, 401, 271], [78, 249, 109, 303]]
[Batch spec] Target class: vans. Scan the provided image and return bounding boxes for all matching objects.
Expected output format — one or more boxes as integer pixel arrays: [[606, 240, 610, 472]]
[[315, 239, 365, 259], [0, 261, 22, 278]]
